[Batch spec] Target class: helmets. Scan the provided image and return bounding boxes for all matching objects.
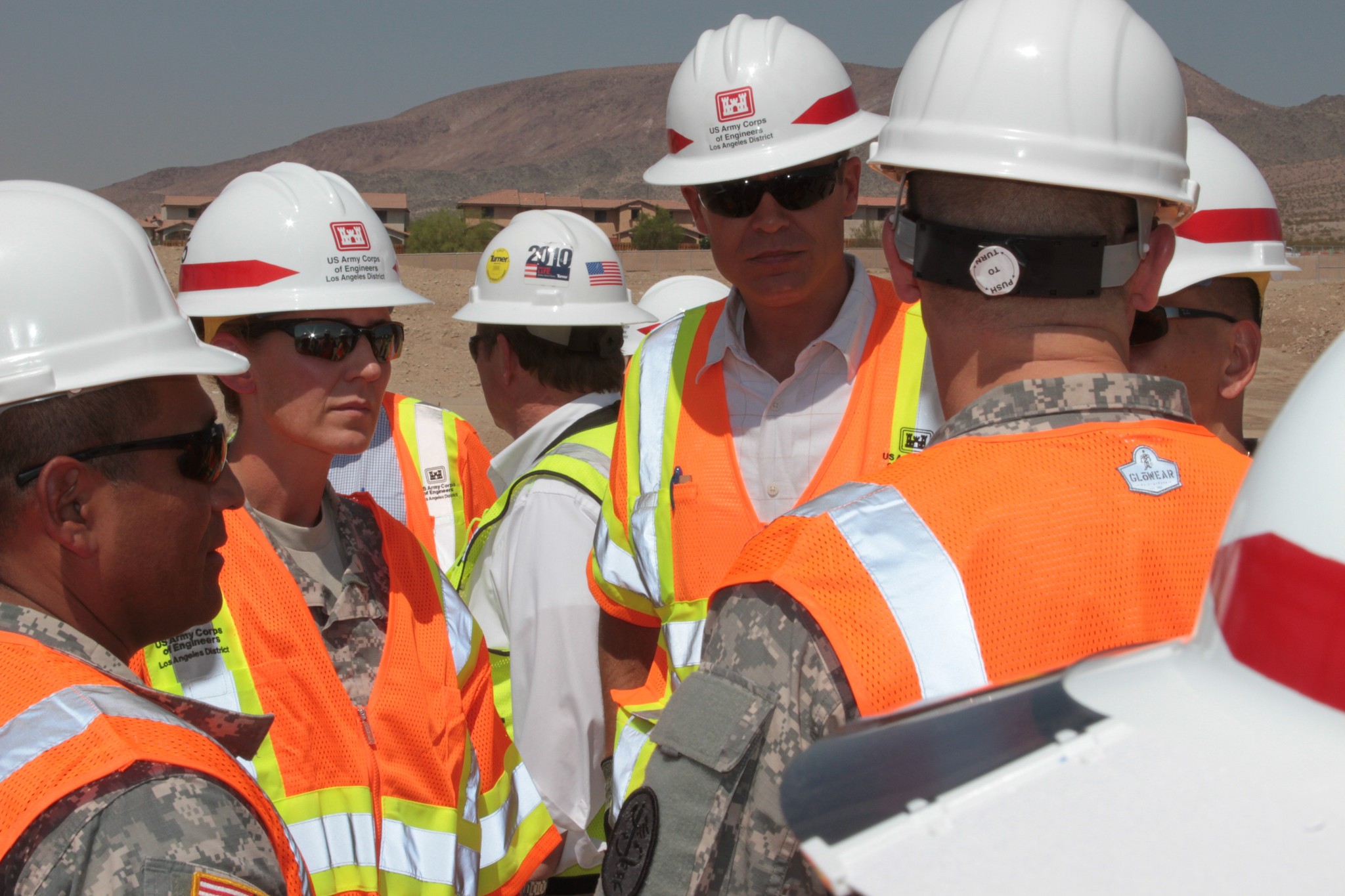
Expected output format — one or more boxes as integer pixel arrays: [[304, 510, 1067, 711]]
[[179, 161, 437, 319], [780, 329, 1345, 896], [618, 276, 730, 357], [1153, 116, 1302, 299], [642, 13, 889, 186], [0, 179, 252, 416], [451, 210, 663, 328], [867, 0, 1198, 296]]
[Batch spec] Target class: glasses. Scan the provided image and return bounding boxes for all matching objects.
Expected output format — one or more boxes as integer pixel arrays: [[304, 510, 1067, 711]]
[[1124, 306, 1236, 346], [465, 334, 492, 363], [13, 425, 231, 492], [689, 158, 848, 218], [249, 320, 406, 363]]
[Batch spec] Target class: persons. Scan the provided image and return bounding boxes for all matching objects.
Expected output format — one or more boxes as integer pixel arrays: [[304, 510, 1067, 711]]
[[585, 1, 1251, 894], [1109, 99, 1286, 464], [3, 176, 329, 895], [583, 3, 948, 831], [124, 159, 564, 896], [440, 212, 682, 896], [319, 368, 504, 593]]
